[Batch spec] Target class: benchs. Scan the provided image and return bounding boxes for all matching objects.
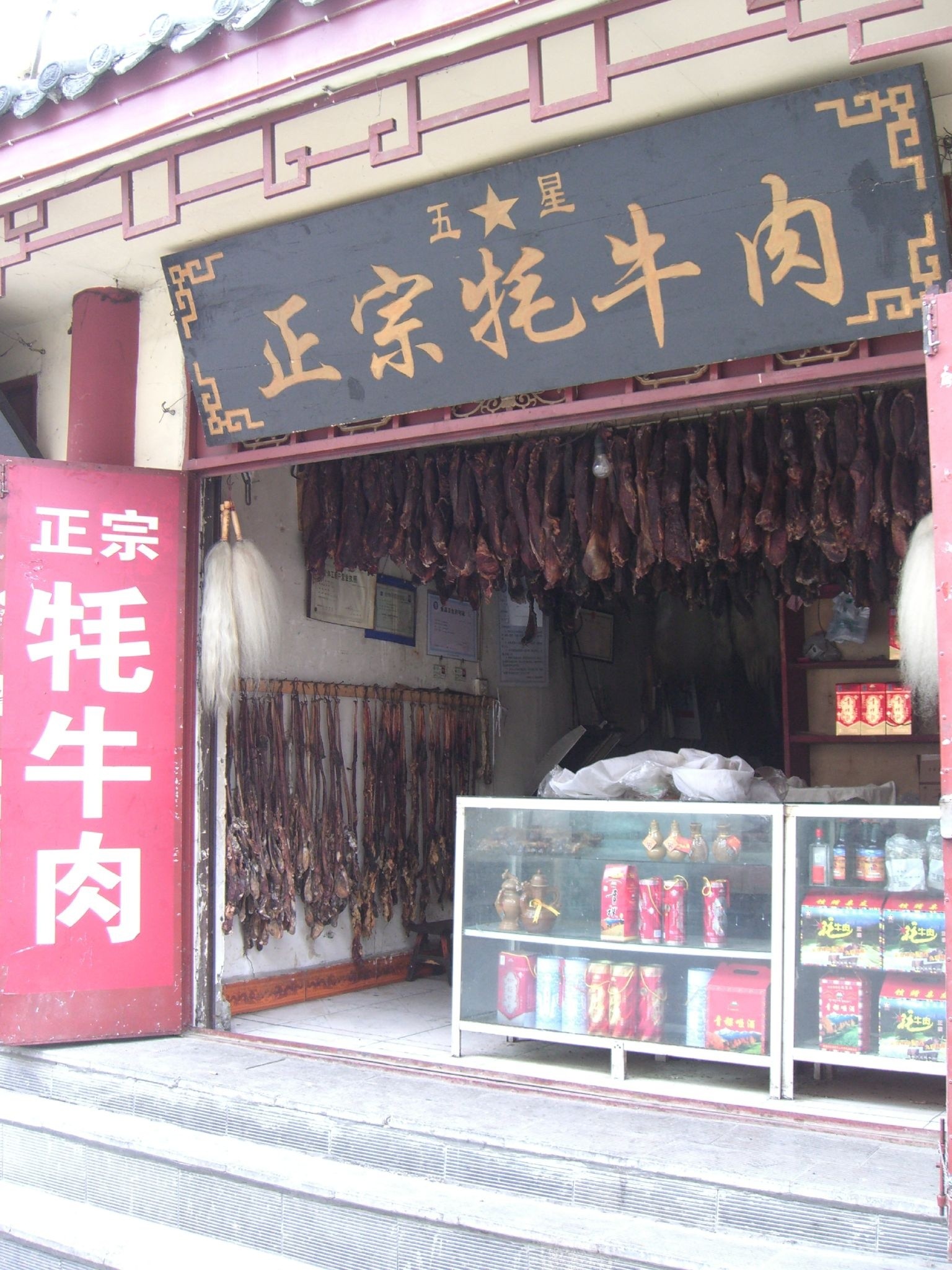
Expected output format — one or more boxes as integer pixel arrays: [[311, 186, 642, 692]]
[[408, 920, 453, 985]]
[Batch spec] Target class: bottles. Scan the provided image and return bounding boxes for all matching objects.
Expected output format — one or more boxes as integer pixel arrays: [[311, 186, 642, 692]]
[[647, 819, 740, 864], [808, 816, 888, 889]]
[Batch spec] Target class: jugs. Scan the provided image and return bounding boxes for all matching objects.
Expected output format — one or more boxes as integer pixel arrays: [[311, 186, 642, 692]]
[[495, 880, 521, 929], [522, 871, 559, 934]]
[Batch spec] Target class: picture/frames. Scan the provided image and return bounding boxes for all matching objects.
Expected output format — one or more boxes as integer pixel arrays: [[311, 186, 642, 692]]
[[569, 608, 616, 662]]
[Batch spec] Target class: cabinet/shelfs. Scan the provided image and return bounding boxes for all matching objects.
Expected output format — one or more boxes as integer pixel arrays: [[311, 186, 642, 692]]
[[784, 806, 946, 1100], [446, 793, 787, 1097], [781, 583, 939, 793]]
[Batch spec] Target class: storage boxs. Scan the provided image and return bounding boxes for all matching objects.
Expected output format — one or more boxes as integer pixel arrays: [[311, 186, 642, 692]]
[[836, 682, 918, 735], [706, 963, 780, 1056], [884, 897, 946, 972], [799, 889, 883, 969], [877, 975, 949, 1066], [889, 608, 908, 659], [819, 978, 871, 1056], [492, 948, 542, 1029]]
